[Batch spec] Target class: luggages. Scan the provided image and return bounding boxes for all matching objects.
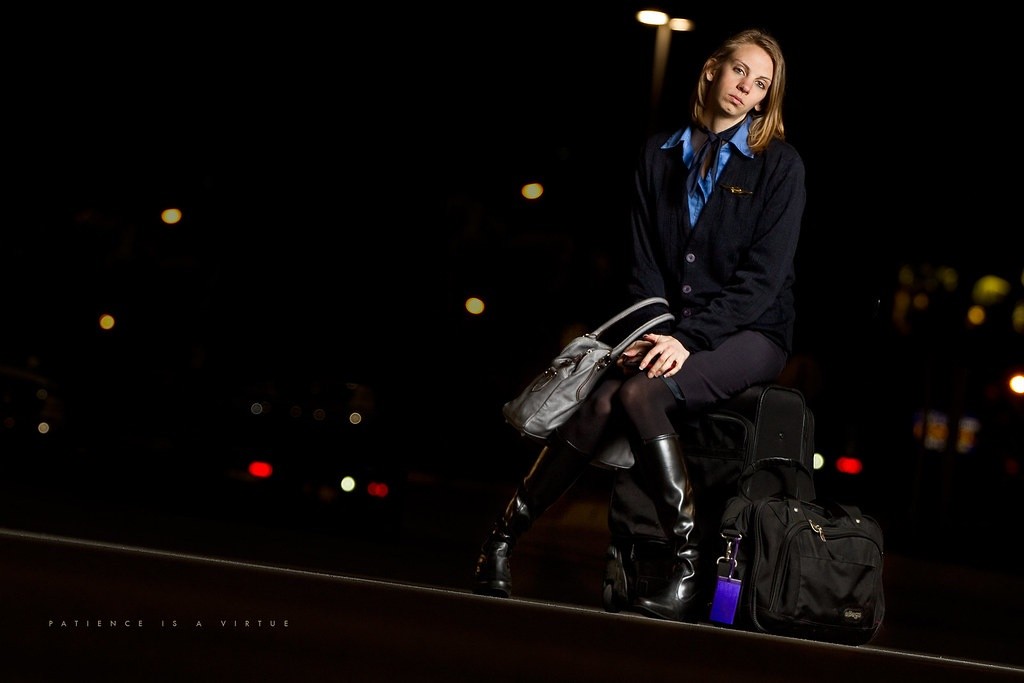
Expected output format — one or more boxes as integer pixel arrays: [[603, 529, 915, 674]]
[[601, 383, 817, 621]]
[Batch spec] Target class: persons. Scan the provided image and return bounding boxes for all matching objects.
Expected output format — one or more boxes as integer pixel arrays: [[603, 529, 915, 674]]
[[472, 26, 807, 623]]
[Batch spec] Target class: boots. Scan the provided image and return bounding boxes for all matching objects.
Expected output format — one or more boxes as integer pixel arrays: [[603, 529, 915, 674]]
[[468, 433, 596, 597], [630, 435, 710, 622]]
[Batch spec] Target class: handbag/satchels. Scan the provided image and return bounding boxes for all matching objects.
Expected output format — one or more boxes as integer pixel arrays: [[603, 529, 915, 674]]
[[504, 297, 676, 438], [712, 458, 885, 645]]
